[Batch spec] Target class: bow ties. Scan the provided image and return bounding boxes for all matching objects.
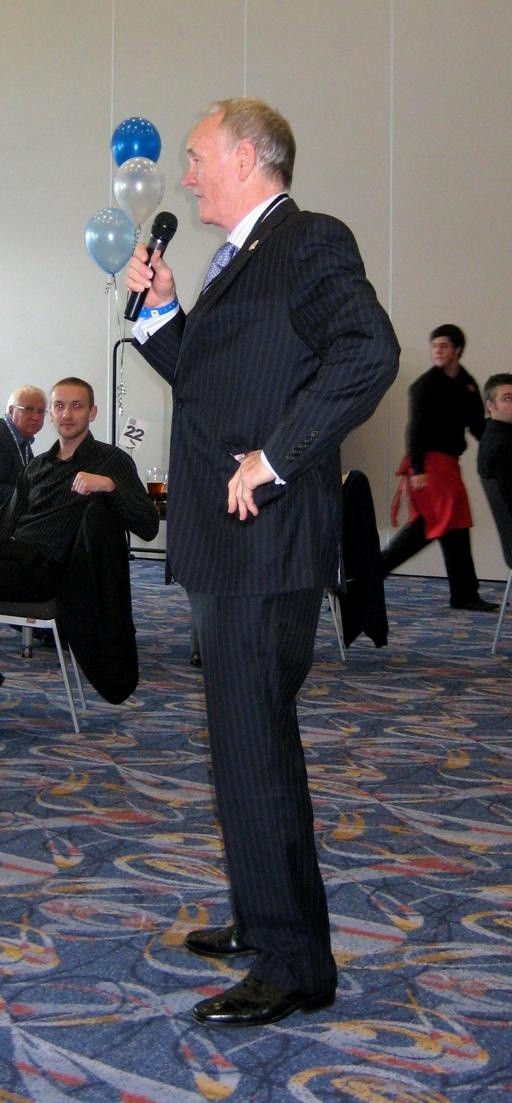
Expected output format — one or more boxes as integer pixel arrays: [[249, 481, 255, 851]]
[[466, 382, 477, 393]]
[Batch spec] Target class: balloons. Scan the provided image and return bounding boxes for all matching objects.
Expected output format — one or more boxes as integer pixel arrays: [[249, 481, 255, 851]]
[[113, 155, 165, 228], [112, 117, 161, 167], [83, 207, 138, 275]]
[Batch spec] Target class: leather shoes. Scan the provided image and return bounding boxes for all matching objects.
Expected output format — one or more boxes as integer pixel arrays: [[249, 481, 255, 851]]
[[190, 971, 338, 1028], [448, 590, 501, 612], [184, 921, 259, 959]]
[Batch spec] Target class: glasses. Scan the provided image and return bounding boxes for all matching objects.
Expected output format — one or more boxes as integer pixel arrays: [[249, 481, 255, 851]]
[[14, 405, 49, 415]]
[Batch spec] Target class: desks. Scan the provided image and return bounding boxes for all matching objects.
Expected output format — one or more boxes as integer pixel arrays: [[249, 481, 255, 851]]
[[126, 492, 170, 562]]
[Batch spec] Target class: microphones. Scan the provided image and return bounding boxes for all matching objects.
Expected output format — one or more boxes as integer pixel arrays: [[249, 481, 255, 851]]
[[121, 214, 176, 321]]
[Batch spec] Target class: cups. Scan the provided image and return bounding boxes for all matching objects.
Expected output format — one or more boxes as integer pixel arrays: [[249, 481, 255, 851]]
[[144, 466, 168, 497]]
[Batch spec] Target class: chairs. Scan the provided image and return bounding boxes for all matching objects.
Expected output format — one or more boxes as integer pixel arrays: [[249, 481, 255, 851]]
[[480, 475, 512, 656], [0, 487, 130, 732], [327, 470, 362, 663]]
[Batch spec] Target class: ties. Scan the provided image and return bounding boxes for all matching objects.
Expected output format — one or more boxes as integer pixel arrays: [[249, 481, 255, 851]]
[[200, 241, 242, 292]]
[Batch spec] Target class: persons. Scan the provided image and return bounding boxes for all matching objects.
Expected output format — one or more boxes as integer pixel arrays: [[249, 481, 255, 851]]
[[379, 324, 500, 610], [475, 371, 512, 516], [0, 385, 49, 509], [122, 95, 401, 1027], [0, 376, 159, 705]]
